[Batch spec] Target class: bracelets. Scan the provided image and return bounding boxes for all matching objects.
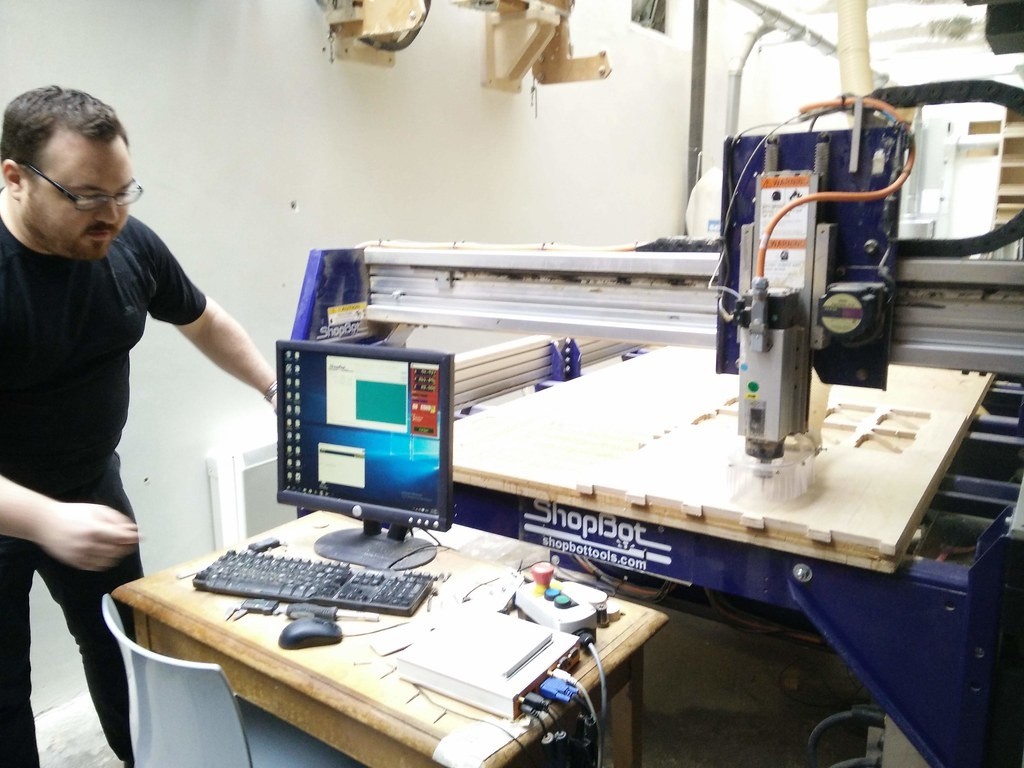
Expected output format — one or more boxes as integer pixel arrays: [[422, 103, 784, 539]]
[[263, 379, 277, 403]]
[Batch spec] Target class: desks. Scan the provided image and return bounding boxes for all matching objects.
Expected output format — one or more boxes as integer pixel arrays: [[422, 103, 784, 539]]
[[113, 509, 673, 768]]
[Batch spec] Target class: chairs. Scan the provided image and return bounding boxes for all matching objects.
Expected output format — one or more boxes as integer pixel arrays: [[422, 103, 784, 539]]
[[101, 592, 367, 768]]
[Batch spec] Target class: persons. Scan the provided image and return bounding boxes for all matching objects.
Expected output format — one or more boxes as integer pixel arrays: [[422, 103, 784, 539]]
[[1, 84, 277, 768]]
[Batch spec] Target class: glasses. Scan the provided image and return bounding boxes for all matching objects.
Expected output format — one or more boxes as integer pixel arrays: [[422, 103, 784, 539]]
[[18, 160, 143, 211]]
